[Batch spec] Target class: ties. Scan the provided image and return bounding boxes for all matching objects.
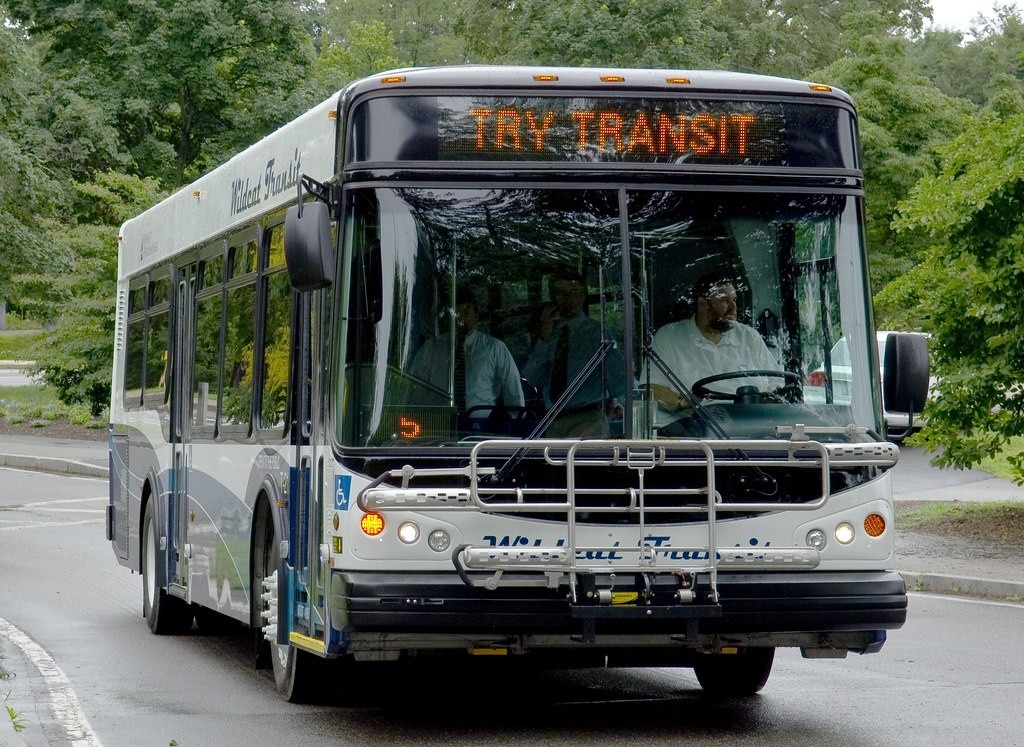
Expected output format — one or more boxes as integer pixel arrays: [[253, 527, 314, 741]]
[[455, 336, 467, 417], [551, 324, 570, 390]]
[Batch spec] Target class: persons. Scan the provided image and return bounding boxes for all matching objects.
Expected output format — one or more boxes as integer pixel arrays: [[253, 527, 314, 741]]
[[639, 266, 787, 435], [408, 289, 525, 431], [522, 270, 627, 441]]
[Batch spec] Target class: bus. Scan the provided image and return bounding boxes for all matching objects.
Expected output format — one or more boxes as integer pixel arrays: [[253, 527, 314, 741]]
[[106, 65, 931, 710]]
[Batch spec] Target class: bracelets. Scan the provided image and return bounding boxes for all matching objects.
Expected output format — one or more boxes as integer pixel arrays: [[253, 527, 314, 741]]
[[677, 393, 684, 410]]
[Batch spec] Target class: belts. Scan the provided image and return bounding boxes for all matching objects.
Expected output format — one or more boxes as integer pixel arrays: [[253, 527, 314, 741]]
[[552, 402, 602, 420]]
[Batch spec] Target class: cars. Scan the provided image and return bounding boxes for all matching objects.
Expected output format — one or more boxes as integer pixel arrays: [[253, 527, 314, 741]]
[[800, 328, 1024, 437]]
